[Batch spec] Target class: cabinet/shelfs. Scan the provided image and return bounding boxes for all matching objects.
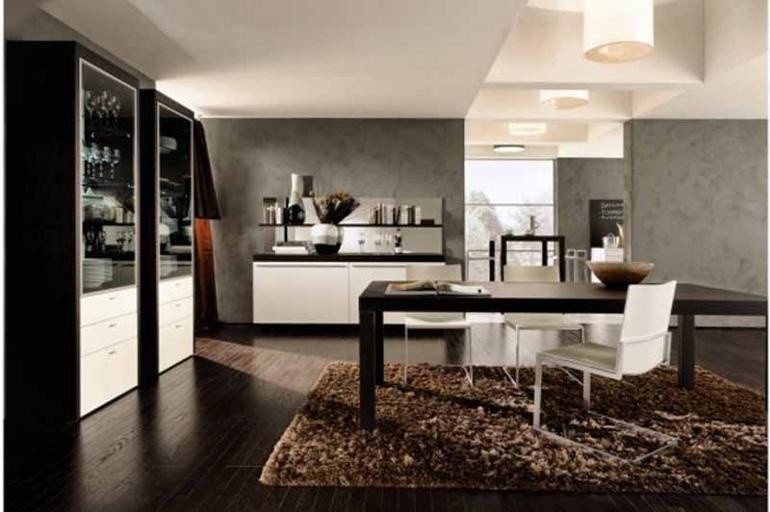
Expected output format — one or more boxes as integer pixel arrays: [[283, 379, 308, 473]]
[[5, 39, 139, 429], [139, 88, 196, 381], [349, 263, 446, 324], [252, 260, 348, 326]]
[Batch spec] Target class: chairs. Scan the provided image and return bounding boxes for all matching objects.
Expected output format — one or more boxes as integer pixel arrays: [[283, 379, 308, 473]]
[[531, 280, 680, 467], [500, 234, 567, 281], [503, 263, 592, 390], [403, 265, 479, 390]]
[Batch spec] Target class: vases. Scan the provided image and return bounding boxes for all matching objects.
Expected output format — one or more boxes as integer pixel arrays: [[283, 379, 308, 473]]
[[336, 225, 344, 250], [288, 172, 305, 224], [310, 223, 338, 255]]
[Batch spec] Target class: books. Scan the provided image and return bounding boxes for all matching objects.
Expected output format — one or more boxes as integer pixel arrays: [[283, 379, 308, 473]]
[[384, 278, 492, 298], [272, 241, 307, 251], [275, 251, 309, 255], [366, 203, 422, 225]]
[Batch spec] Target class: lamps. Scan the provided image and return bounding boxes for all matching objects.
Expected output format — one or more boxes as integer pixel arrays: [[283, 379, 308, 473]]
[[540, 90, 591, 110], [583, 0, 654, 63], [508, 123, 548, 137]]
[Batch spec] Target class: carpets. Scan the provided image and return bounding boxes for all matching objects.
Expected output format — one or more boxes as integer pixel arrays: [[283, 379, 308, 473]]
[[259, 362, 767, 495]]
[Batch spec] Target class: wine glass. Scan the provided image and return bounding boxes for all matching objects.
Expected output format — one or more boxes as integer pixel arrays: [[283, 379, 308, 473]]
[[83, 143, 121, 178], [101, 89, 112, 118], [110, 95, 120, 118], [85, 90, 95, 118], [95, 93, 104, 118]]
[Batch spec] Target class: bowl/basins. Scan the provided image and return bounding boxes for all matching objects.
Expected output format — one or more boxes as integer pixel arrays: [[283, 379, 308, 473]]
[[82, 259, 105, 288], [586, 261, 654, 289]]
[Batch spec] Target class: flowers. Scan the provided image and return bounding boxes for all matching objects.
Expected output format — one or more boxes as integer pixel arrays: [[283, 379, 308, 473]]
[[308, 191, 359, 224]]
